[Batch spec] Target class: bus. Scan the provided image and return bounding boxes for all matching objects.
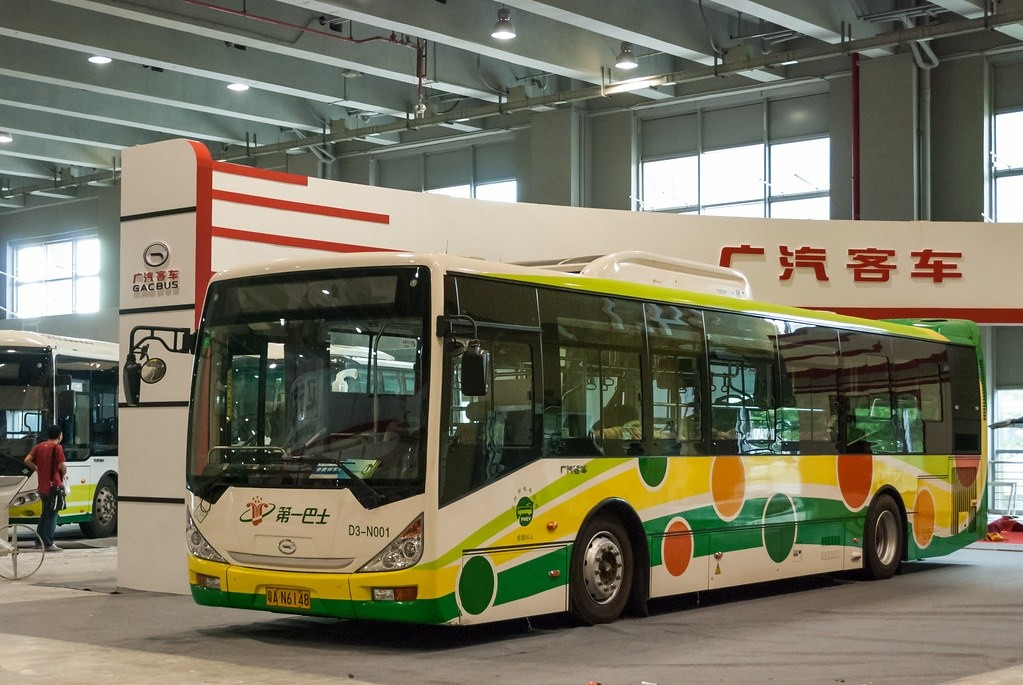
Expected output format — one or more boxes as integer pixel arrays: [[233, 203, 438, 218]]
[[122, 250, 987, 626], [0, 328, 119, 541]]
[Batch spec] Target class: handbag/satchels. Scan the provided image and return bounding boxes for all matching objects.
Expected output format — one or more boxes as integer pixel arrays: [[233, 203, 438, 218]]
[[48, 484, 66, 512], [62, 476, 71, 496]]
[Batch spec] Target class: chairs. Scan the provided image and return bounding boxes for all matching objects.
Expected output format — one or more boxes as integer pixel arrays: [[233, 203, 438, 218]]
[[457, 400, 737, 448], [94, 417, 118, 442]]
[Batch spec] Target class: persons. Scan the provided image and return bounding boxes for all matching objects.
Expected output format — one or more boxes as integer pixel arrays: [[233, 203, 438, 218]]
[[24, 424, 68, 553], [595, 405, 739, 439]]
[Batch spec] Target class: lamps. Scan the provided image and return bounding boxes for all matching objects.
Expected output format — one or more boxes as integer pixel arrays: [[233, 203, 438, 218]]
[[614, 39, 638, 69], [0, 131, 13, 142], [491, 2, 516, 40]]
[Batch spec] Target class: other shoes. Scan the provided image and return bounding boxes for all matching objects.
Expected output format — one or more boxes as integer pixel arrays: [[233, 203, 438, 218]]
[[34, 540, 43, 549], [45, 543, 63, 552]]
